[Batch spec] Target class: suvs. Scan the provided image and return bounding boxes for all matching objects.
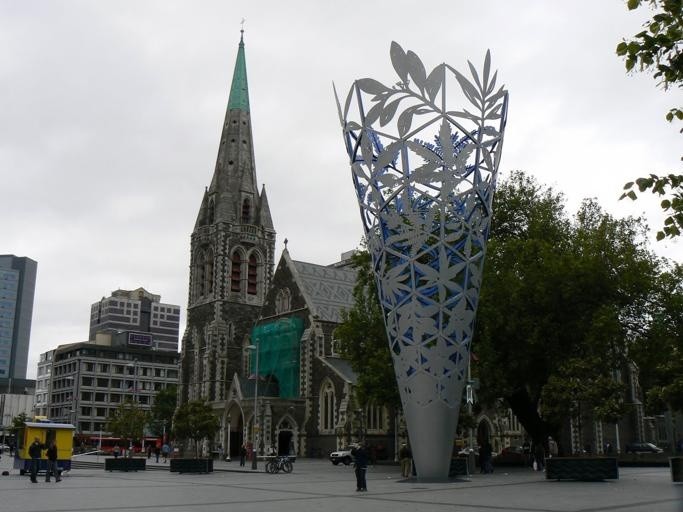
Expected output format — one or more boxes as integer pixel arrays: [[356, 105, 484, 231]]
[[329, 444, 356, 466]]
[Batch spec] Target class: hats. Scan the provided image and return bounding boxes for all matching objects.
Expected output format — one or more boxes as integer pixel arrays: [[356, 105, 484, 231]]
[[548, 436, 552, 439]]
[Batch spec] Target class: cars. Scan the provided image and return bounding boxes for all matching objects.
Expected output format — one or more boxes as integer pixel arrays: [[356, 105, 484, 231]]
[[624, 443, 666, 455], [0, 443, 16, 452]]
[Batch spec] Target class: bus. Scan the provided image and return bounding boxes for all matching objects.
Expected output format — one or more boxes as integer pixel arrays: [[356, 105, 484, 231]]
[[86, 437, 158, 455]]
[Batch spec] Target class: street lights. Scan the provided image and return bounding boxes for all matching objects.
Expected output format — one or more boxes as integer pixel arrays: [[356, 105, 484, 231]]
[[225, 413, 232, 462], [245, 337, 266, 470]]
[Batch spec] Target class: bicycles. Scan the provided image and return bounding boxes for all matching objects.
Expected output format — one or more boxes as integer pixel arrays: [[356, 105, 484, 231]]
[[265, 454, 293, 474]]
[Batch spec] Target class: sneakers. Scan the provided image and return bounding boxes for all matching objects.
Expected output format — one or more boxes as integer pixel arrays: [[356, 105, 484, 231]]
[[30, 477, 61, 482], [356, 487, 366, 491]]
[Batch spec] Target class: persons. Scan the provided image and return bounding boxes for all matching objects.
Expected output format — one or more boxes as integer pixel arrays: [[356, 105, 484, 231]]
[[114, 443, 120, 459], [484, 443, 494, 474], [28, 436, 46, 483], [10, 443, 13, 456], [239, 444, 246, 467], [399, 443, 410, 477], [535, 443, 545, 471], [45, 439, 61, 482], [246, 441, 253, 461], [548, 436, 559, 458], [218, 446, 225, 461], [522, 438, 530, 455], [350, 441, 368, 491], [147, 444, 152, 458], [478, 443, 484, 474], [162, 443, 170, 463], [155, 445, 161, 463]]
